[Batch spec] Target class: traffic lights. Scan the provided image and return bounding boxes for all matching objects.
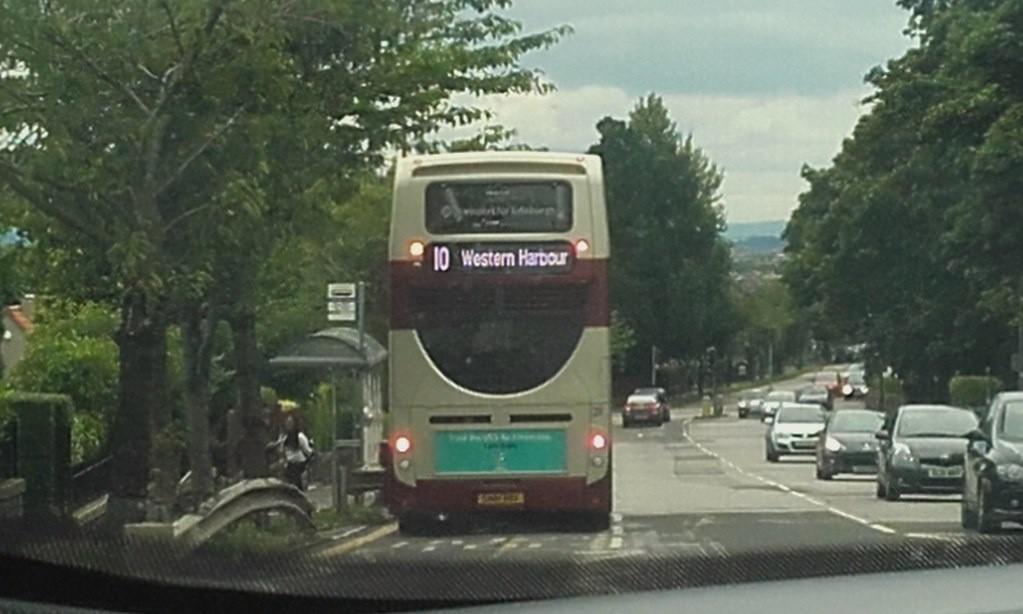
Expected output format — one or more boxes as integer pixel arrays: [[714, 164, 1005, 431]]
[[706, 347, 715, 367]]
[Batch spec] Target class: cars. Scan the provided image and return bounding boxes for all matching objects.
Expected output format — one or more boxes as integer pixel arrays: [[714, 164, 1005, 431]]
[[815, 410, 886, 480], [622, 394, 664, 426], [874, 406, 979, 501], [842, 364, 870, 401], [812, 371, 842, 397], [961, 392, 1023, 535], [737, 392, 764, 418], [634, 387, 671, 422], [763, 403, 826, 461], [795, 384, 833, 410], [760, 391, 796, 423]]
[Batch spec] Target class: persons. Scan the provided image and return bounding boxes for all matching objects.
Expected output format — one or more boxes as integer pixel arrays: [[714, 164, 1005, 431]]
[[832, 372, 852, 398], [277, 413, 313, 490], [234, 418, 277, 480]]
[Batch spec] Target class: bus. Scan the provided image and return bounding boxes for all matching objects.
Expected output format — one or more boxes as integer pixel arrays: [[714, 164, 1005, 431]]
[[378, 151, 613, 532]]
